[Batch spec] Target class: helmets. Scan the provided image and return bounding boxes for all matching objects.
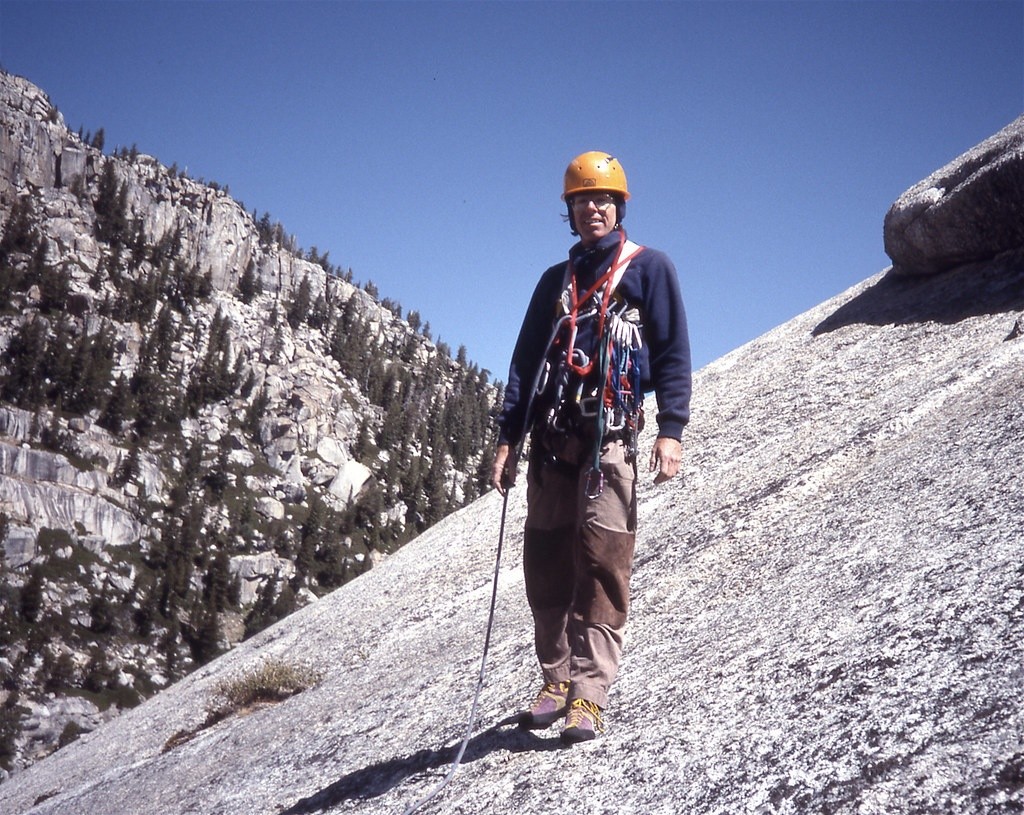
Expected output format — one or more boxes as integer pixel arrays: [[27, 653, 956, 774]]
[[561, 150, 631, 200]]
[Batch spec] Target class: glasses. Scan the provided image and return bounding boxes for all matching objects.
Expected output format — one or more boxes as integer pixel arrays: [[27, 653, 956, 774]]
[[572, 194, 616, 212]]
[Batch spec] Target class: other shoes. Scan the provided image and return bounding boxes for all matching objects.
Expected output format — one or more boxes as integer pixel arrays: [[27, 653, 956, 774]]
[[518, 680, 571, 729], [561, 698, 604, 742]]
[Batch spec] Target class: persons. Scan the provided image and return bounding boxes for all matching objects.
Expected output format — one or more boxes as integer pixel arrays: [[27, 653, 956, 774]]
[[491, 151, 693, 743]]
